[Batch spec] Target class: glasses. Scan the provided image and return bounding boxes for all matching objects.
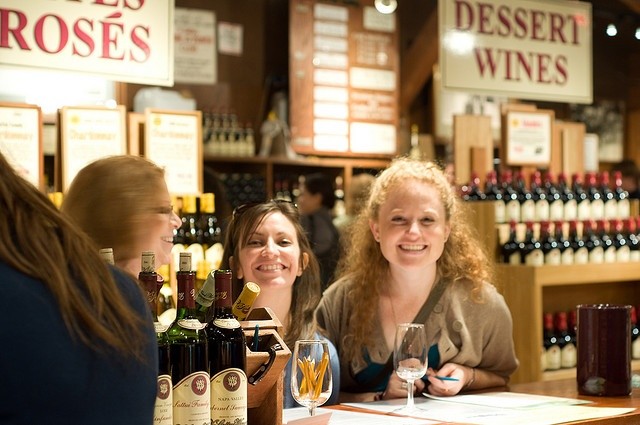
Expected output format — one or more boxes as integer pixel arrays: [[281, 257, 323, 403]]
[[232, 199, 293, 218]]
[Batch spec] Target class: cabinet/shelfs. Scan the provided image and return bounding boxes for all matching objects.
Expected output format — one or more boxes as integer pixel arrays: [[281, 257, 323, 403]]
[[451, 116, 640, 381], [204, 157, 389, 222]]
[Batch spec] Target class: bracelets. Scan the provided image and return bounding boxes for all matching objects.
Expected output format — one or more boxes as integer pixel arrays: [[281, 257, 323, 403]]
[[464, 368, 479, 393]]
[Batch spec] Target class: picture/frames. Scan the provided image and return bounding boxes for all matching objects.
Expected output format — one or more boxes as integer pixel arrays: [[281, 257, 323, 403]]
[[0, 102, 44, 196], [142, 109, 204, 195], [59, 105, 130, 188]]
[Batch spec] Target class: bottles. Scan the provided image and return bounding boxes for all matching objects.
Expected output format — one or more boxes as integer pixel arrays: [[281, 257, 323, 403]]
[[522, 222, 544, 266], [197, 193, 220, 250], [550, 171, 562, 221], [459, 183, 473, 202], [564, 174, 577, 221], [179, 194, 196, 249], [191, 269, 228, 313], [167, 193, 180, 244], [233, 281, 261, 319], [589, 222, 604, 264], [528, 170, 549, 224], [624, 305, 640, 360], [542, 311, 560, 370], [482, 171, 505, 223], [200, 106, 256, 161], [513, 169, 535, 223], [591, 171, 603, 220], [502, 223, 527, 265], [631, 218, 640, 262], [409, 125, 421, 160], [602, 220, 617, 262], [556, 312, 577, 368], [570, 311, 577, 345], [547, 222, 561, 265], [614, 172, 630, 219], [138, 272, 173, 425], [497, 168, 522, 224], [614, 220, 631, 262], [207, 270, 248, 424], [600, 171, 618, 219], [577, 174, 590, 219], [164, 270, 211, 425], [465, 173, 486, 224], [48, 193, 63, 208], [558, 222, 574, 264], [572, 222, 588, 263]]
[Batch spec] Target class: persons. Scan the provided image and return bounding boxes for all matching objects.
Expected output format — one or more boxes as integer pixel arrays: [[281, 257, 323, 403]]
[[199, 198, 343, 411], [1, 149, 162, 425], [61, 153, 185, 289], [310, 155, 520, 404]]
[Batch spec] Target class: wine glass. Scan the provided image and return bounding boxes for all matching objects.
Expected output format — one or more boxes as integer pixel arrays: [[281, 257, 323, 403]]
[[291, 341, 333, 418], [393, 324, 429, 416]]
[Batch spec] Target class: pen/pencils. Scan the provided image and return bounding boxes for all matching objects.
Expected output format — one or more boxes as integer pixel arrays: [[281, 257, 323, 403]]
[[422, 375, 459, 381], [254, 325, 259, 351]]
